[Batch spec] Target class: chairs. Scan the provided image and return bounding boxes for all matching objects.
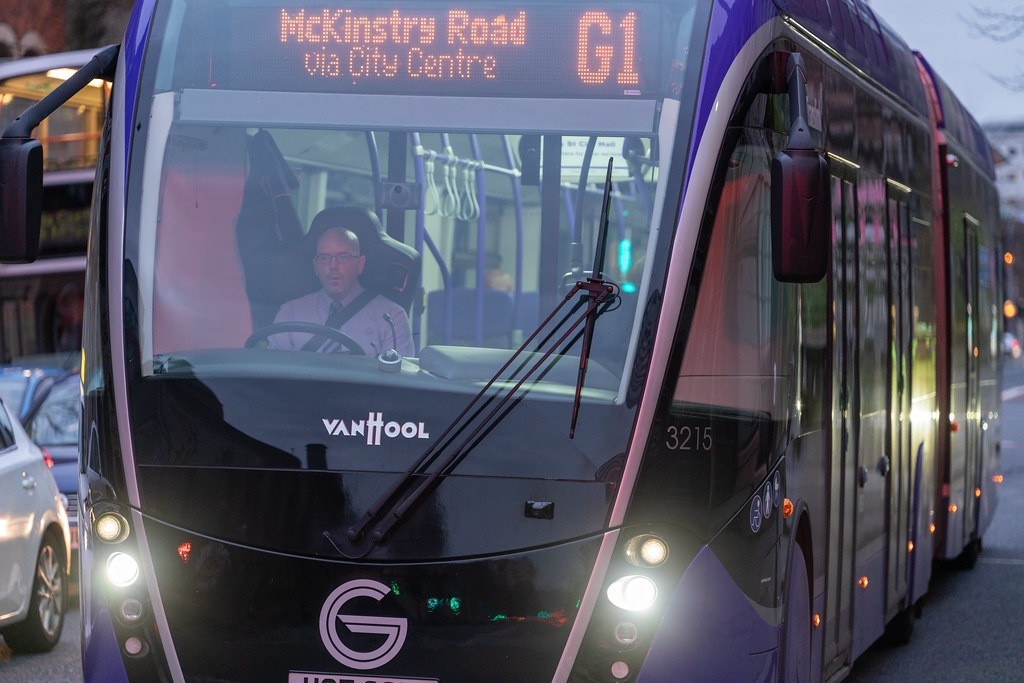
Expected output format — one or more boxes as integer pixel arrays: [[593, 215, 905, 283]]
[[514, 291, 540, 338], [425, 289, 514, 351], [287, 203, 423, 314]]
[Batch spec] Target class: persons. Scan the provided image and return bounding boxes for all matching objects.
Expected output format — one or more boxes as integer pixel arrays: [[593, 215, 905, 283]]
[[263, 223, 415, 357]]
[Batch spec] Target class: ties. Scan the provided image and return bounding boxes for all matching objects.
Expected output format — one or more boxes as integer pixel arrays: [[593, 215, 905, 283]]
[[322, 301, 345, 353]]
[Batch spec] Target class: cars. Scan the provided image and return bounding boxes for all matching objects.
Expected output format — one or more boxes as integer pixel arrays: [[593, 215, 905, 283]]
[[0, 396, 79, 655], [16, 369, 81, 575], [0, 348, 82, 418]]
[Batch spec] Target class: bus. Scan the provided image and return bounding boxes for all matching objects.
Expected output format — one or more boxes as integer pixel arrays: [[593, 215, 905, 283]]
[[0, 0, 1007, 683]]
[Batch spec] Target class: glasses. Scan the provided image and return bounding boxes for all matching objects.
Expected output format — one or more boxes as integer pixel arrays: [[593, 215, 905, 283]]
[[317, 252, 360, 264]]
